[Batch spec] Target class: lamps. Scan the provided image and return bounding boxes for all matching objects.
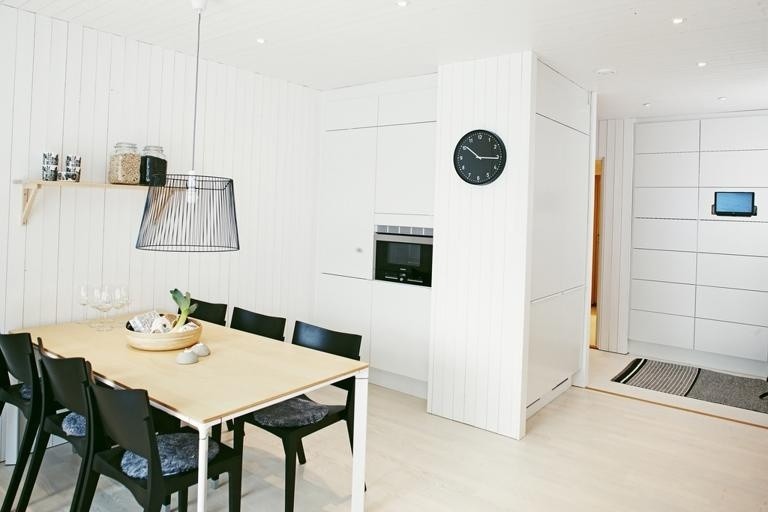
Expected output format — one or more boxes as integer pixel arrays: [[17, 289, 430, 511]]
[[133, 1, 242, 253]]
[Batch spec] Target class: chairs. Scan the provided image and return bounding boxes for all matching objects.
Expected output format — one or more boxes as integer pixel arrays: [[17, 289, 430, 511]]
[[0, 299, 369, 512]]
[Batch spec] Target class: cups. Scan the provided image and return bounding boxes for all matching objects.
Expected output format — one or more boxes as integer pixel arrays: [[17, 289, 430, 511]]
[[39, 151, 60, 181], [56, 154, 81, 182]]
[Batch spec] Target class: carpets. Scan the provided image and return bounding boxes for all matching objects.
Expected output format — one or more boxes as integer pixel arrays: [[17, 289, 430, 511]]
[[609, 357, 767, 415]]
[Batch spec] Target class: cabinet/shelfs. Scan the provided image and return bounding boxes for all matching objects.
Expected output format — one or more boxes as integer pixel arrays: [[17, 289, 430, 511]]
[[312, 72, 436, 384]]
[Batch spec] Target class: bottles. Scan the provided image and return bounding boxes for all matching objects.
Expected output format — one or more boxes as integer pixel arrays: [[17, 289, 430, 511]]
[[106, 141, 168, 187]]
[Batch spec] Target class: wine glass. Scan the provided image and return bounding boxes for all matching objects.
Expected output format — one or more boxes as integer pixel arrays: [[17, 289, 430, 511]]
[[75, 283, 129, 332]]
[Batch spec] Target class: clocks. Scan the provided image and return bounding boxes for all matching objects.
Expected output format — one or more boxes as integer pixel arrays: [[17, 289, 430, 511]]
[[454, 127, 507, 185]]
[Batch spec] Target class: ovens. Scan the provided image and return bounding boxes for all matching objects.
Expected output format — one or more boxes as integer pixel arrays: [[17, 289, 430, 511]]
[[371, 229, 434, 289]]
